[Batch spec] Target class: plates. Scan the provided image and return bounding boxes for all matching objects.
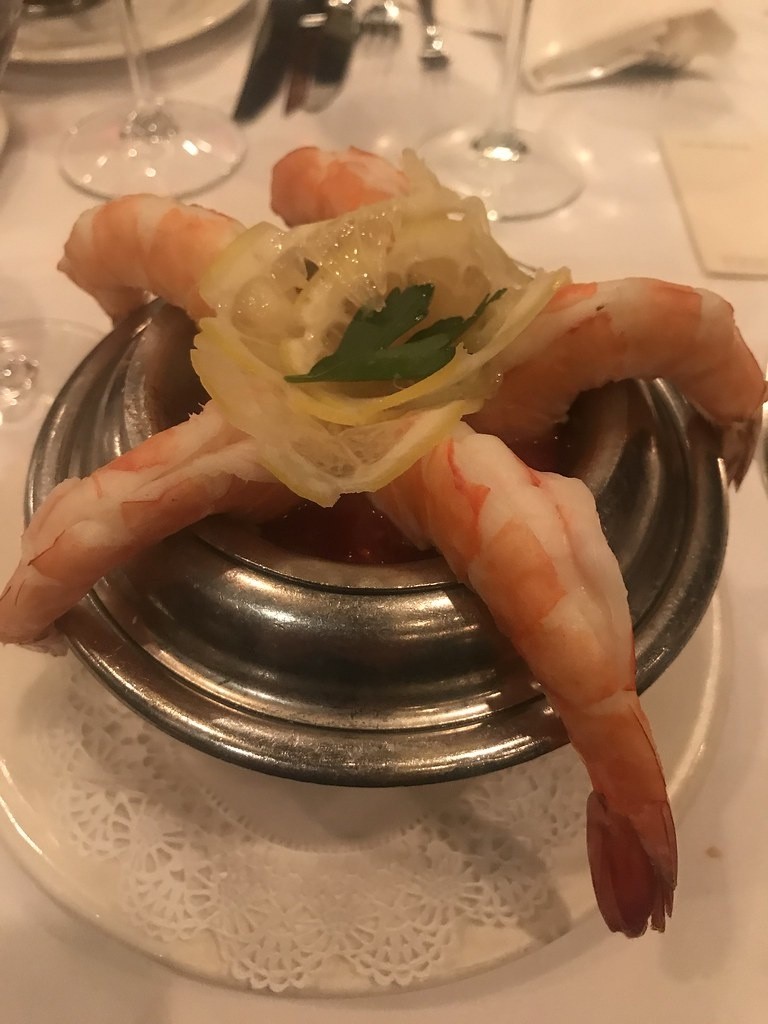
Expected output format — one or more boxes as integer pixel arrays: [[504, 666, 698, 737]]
[[8, 0, 252, 64], [2, 487, 726, 995]]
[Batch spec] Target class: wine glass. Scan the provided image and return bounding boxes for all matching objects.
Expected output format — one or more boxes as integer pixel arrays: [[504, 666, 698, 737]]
[[62, 1, 249, 198], [2, 319, 110, 450], [1, 0, 25, 152], [416, 0, 588, 218]]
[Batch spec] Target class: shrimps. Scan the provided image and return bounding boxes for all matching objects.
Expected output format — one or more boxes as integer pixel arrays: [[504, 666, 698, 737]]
[[269, 141, 408, 228], [55, 193, 250, 332], [0, 399, 308, 659], [365, 420, 680, 939], [460, 276, 768, 492]]
